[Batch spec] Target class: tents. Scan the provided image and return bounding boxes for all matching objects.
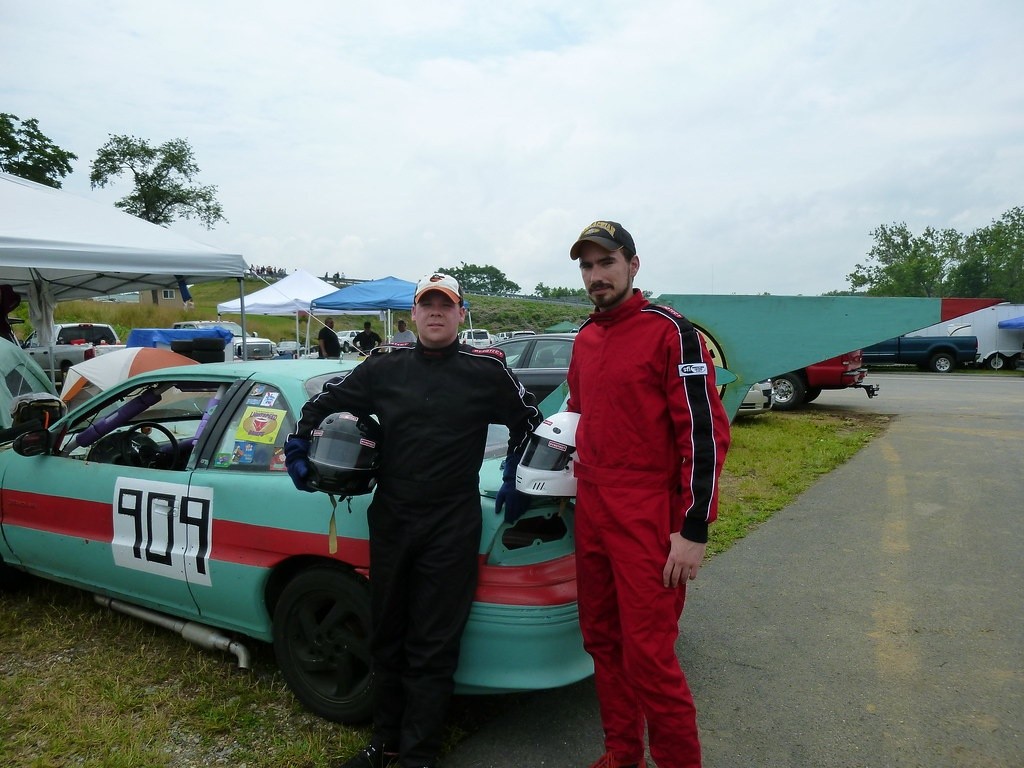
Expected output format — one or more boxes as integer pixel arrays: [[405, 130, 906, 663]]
[[0, 163, 367, 423], [215, 271, 398, 362]]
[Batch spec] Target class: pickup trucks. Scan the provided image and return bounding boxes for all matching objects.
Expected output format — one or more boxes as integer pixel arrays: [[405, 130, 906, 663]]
[[860, 332, 979, 373]]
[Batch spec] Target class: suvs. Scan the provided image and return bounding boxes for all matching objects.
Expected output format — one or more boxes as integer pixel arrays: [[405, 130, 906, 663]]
[[735, 348, 879, 418], [458, 326, 581, 408], [0, 319, 378, 445]]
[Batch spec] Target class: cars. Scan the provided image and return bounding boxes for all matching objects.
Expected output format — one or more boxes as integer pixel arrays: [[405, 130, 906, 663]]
[[0, 295, 941, 728]]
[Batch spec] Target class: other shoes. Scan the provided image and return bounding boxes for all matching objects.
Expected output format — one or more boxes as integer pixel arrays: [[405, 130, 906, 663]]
[[338, 742, 399, 768]]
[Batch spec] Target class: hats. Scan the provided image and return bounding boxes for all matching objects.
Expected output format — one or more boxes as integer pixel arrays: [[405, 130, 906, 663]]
[[414, 272, 463, 304], [364, 322, 371, 327], [570, 221, 636, 260]]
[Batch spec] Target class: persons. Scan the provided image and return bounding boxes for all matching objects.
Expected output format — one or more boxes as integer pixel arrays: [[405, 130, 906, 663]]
[[390, 320, 418, 343], [283, 271, 546, 768], [565, 220, 731, 768], [353, 321, 383, 357], [316, 317, 341, 360]]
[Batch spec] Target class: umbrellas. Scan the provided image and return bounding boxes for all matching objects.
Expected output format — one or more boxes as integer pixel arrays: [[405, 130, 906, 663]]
[[309, 273, 472, 359]]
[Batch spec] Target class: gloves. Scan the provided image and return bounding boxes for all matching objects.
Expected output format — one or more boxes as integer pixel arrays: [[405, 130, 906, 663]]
[[495, 455, 531, 524], [284, 438, 311, 490]]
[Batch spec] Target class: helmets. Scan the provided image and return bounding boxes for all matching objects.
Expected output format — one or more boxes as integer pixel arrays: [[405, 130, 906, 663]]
[[515, 412, 581, 496], [305, 409, 384, 495]]
